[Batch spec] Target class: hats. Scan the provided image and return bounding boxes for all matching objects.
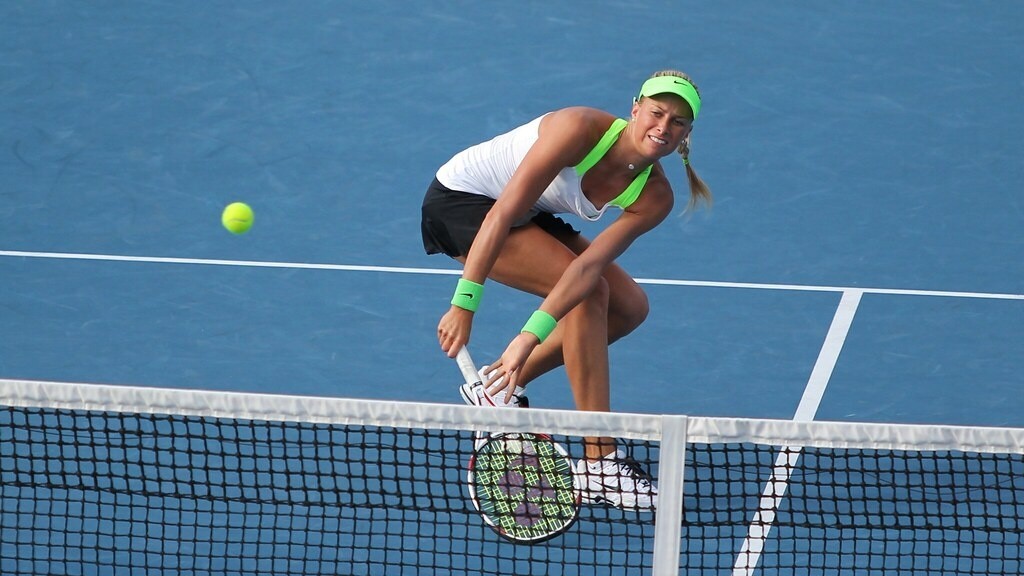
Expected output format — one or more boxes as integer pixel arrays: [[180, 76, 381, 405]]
[[639, 76, 702, 122]]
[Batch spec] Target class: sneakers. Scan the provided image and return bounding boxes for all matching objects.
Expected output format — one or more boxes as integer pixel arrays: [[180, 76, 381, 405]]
[[459, 364, 527, 452], [572, 448, 659, 512]]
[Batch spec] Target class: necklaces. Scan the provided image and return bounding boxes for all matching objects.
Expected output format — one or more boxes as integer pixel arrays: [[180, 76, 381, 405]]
[[616, 134, 651, 172]]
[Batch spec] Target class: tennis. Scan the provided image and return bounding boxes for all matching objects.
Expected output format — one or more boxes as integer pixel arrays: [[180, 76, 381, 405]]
[[220, 201, 255, 235]]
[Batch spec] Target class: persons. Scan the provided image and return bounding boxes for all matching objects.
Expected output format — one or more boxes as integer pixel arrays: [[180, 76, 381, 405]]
[[417, 68, 703, 513]]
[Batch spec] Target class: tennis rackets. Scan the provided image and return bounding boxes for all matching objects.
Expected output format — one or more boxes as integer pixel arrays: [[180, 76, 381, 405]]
[[455, 344, 585, 546]]
